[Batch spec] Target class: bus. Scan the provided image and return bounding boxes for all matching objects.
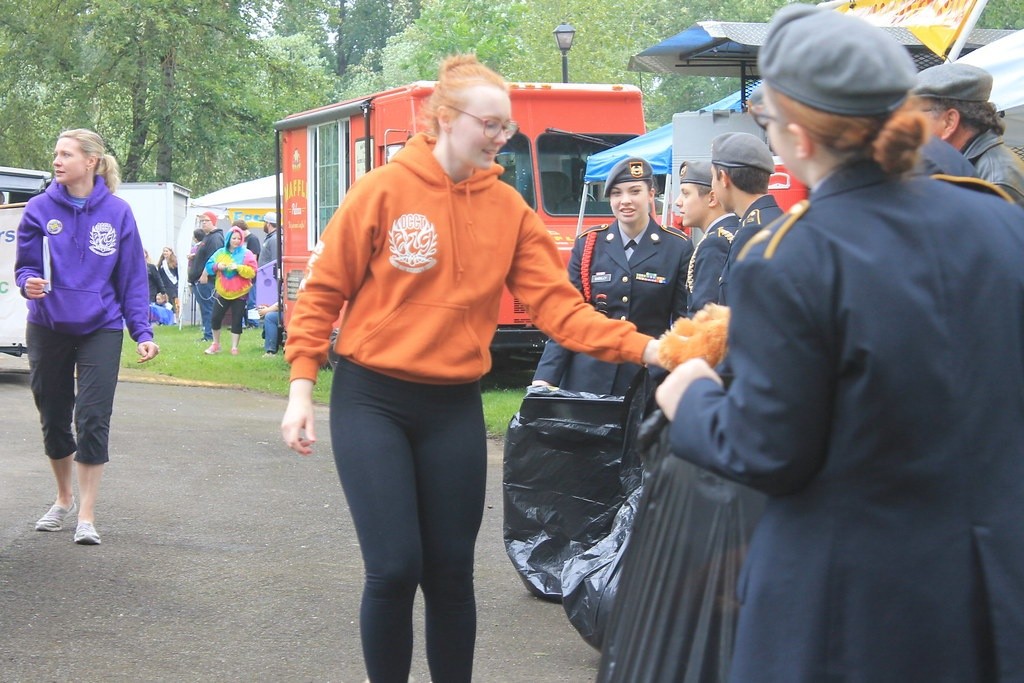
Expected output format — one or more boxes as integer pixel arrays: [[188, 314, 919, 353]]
[[272, 83, 691, 391]]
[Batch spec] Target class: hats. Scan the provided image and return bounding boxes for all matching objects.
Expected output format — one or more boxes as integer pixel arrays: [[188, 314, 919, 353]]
[[711, 132, 776, 175], [757, 3, 916, 115], [264, 212, 276, 223], [604, 156, 654, 198], [912, 63, 993, 102], [679, 160, 713, 186], [204, 212, 217, 226]]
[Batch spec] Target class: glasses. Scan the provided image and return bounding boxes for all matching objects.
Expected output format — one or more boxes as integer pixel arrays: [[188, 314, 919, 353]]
[[447, 105, 519, 140], [200, 219, 212, 222]]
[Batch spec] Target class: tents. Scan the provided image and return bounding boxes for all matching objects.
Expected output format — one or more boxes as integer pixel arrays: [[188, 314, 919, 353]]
[[575, 80, 762, 233], [953, 28, 1024, 147], [178, 174, 281, 331]]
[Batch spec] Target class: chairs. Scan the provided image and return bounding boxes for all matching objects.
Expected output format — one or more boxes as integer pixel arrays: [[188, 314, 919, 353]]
[[529, 171, 583, 213]]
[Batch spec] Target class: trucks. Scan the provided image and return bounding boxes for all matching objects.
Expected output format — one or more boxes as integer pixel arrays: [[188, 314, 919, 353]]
[[115, 182, 192, 269], [0, 164, 53, 375]]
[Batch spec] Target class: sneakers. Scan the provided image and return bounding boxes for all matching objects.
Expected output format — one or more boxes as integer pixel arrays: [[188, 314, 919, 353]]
[[35, 495, 77, 531], [74, 521, 102, 544]]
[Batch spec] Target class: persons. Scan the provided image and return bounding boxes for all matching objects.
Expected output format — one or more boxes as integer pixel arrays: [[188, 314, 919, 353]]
[[532, 5, 1024, 683], [14, 129, 159, 543], [144, 211, 284, 358], [282, 55, 665, 683]]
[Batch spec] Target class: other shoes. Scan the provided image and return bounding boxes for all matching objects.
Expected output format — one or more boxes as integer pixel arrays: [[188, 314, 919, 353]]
[[204, 342, 222, 354], [231, 348, 240, 355], [261, 352, 279, 358], [196, 338, 209, 342]]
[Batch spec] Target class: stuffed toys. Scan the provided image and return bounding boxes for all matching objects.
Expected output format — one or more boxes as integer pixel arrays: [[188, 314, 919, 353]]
[[658, 302, 731, 375]]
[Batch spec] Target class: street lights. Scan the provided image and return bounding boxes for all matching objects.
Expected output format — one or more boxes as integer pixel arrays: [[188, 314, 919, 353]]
[[552, 22, 576, 84]]
